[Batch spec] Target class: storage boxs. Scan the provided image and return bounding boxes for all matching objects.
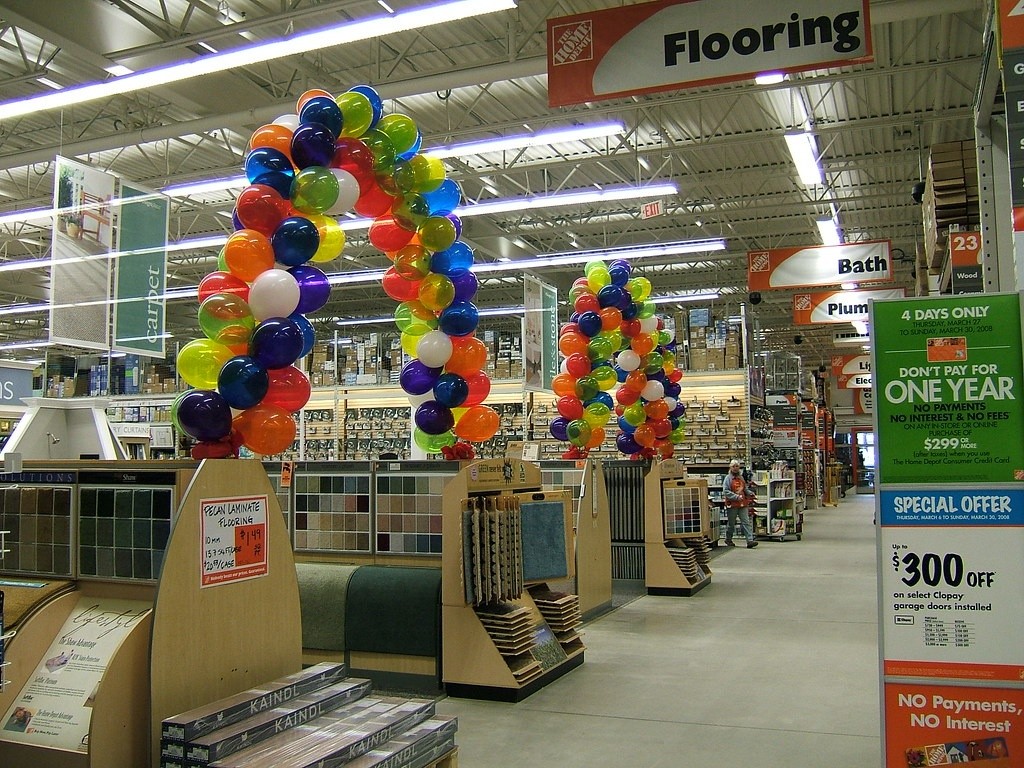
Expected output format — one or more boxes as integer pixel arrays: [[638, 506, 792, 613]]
[[155, 383, 162, 392], [155, 410, 160, 421], [150, 406, 155, 421], [138, 416, 145, 422], [63, 377, 74, 397], [140, 407, 148, 417], [165, 405, 172, 410], [107, 408, 115, 415], [304, 308, 741, 388], [46, 389, 59, 398], [53, 375, 66, 382], [123, 408, 133, 414], [133, 414, 139, 422], [121, 414, 132, 421], [160, 410, 165, 421], [133, 408, 139, 414], [163, 378, 176, 393]]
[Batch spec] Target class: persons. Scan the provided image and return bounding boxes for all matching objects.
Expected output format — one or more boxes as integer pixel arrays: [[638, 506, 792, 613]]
[[722, 459, 759, 548]]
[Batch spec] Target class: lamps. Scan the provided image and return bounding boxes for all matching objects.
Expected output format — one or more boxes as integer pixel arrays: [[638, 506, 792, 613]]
[[817, 214, 843, 246], [783, 127, 824, 185], [0, 0, 730, 351]]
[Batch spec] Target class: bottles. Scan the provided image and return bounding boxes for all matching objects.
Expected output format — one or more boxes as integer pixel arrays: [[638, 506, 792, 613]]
[[772, 463, 789, 479]]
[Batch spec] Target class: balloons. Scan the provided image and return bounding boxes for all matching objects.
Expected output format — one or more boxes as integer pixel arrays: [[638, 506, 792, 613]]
[[170, 86, 500, 455], [549, 258, 686, 458]]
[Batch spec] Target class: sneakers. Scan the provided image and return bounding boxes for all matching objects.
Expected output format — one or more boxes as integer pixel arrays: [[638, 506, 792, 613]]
[[725, 540, 735, 546], [747, 541, 758, 548]]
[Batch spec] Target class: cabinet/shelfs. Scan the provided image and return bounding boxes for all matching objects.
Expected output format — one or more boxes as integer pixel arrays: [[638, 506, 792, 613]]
[[0, 365, 843, 543]]
